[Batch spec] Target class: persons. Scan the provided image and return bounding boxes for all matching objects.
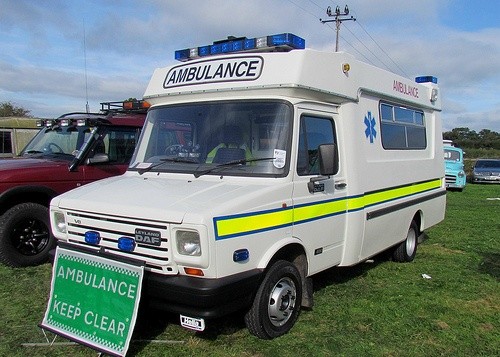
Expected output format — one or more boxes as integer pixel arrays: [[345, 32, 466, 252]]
[[205, 125, 258, 166]]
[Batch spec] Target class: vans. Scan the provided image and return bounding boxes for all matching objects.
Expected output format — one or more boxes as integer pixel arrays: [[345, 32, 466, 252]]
[[50, 31, 447, 338]]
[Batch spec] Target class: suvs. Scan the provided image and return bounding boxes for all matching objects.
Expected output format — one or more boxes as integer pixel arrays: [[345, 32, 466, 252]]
[[0, 98, 147, 264], [443, 146, 466, 192]]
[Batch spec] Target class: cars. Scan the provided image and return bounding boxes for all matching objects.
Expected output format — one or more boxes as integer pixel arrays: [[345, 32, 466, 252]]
[[470, 159, 500, 184]]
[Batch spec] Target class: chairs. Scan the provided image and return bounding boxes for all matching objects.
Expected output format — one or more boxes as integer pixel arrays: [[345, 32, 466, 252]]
[[97, 141, 105, 154], [211, 123, 247, 166]]
